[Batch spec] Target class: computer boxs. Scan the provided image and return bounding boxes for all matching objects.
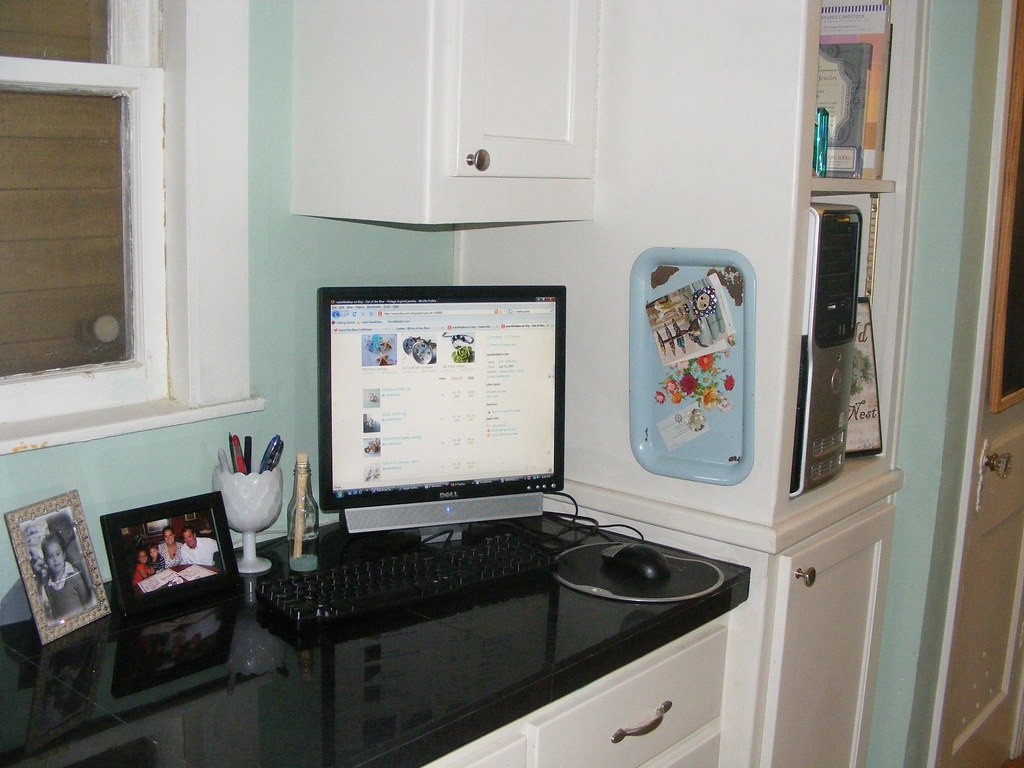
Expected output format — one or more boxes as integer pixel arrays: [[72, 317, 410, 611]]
[[785, 201, 862, 498]]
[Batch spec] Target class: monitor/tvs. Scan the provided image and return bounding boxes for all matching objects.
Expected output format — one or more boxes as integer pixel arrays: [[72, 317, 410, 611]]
[[317, 286, 565, 552]]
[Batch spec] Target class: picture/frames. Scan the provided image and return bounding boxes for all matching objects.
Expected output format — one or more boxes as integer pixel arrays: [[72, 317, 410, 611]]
[[100, 491, 242, 625], [3, 489, 114, 646]]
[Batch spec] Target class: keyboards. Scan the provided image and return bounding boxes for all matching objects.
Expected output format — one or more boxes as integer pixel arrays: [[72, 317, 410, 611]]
[[255, 533, 561, 632]]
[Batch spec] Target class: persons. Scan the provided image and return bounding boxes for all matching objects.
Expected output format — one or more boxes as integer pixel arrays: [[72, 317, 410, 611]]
[[138, 613, 221, 659], [157, 526, 183, 570], [180, 527, 219, 567], [41, 534, 87, 620], [132, 550, 155, 588], [46, 646, 90, 704], [147, 543, 166, 573]]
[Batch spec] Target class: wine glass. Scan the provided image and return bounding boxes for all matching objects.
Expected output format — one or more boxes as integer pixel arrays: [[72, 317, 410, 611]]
[[211, 458, 284, 573]]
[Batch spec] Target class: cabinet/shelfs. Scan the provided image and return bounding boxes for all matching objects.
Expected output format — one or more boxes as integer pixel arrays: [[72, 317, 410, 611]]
[[283, 2, 930, 768]]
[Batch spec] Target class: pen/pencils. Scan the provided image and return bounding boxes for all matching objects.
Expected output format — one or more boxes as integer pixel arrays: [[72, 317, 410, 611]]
[[218, 431, 284, 474]]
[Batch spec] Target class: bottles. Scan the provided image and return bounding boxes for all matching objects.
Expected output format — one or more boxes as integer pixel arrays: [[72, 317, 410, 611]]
[[287, 463, 319, 572]]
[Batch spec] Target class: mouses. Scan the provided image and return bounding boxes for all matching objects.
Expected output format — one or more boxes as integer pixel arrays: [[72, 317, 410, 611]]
[[601, 542, 671, 581]]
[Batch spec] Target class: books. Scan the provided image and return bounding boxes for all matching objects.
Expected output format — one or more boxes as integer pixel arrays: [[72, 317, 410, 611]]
[[844, 298, 884, 456], [816, 4, 894, 181]]
